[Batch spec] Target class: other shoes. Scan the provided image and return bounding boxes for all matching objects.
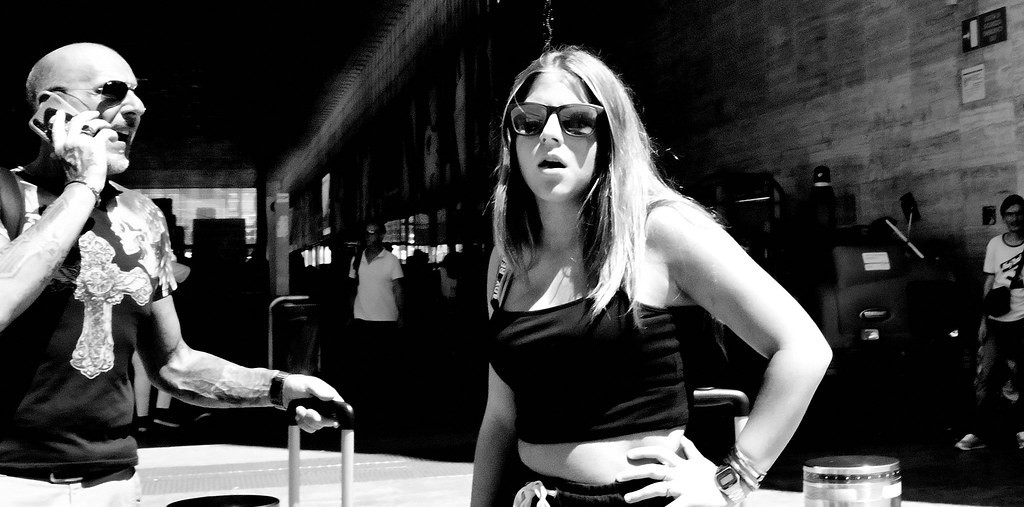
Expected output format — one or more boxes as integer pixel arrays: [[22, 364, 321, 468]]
[[955, 434, 987, 451], [1016, 432, 1024, 448]]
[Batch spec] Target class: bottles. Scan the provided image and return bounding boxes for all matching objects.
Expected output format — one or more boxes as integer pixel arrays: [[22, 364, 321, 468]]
[[802, 455, 902, 507]]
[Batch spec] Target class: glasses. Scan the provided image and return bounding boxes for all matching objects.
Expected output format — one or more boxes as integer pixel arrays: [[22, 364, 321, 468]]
[[509, 100, 604, 137], [52, 80, 142, 102]]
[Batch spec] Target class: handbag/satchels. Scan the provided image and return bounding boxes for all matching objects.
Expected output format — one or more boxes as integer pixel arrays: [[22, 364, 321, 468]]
[[984, 286, 1012, 318]]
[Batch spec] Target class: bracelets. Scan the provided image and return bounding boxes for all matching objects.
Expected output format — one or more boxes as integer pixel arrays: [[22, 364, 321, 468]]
[[64, 179, 101, 209], [268, 371, 290, 409], [722, 443, 768, 491]]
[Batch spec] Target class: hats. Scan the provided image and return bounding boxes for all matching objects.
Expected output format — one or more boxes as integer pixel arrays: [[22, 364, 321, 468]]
[[813, 166, 831, 183]]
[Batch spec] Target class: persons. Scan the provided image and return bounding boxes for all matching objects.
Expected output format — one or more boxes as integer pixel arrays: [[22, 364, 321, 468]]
[[348, 217, 494, 364], [953, 192, 1024, 451], [469, 43, 835, 507], [0, 42, 347, 507]]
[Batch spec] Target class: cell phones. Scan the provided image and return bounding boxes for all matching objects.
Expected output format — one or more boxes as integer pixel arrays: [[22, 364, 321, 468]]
[[29, 94, 90, 146]]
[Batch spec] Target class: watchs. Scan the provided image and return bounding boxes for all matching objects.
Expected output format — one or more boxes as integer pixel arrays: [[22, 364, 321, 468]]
[[715, 464, 746, 503]]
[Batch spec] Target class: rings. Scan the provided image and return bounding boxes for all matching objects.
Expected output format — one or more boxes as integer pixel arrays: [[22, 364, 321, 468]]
[[80, 124, 96, 136]]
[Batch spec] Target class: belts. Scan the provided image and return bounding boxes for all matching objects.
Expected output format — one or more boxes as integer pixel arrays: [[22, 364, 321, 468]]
[[0, 464, 130, 484]]
